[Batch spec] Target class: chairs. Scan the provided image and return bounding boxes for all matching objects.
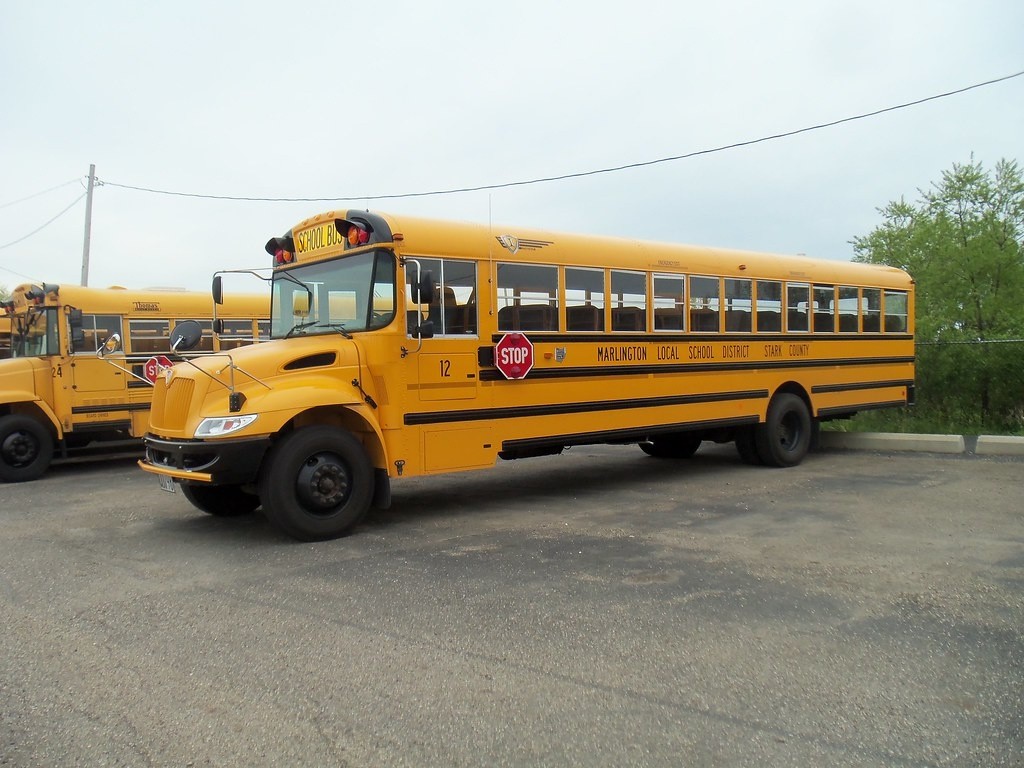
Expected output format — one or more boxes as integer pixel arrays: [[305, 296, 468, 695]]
[[408, 286, 908, 341], [94, 325, 255, 354]]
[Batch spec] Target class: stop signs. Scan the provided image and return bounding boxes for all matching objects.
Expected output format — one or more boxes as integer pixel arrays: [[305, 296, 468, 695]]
[[493, 332, 534, 380], [143, 356, 174, 388]]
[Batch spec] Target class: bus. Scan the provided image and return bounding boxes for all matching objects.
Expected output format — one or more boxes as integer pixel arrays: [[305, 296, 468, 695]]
[[0, 284, 461, 483], [96, 210, 916, 541]]
